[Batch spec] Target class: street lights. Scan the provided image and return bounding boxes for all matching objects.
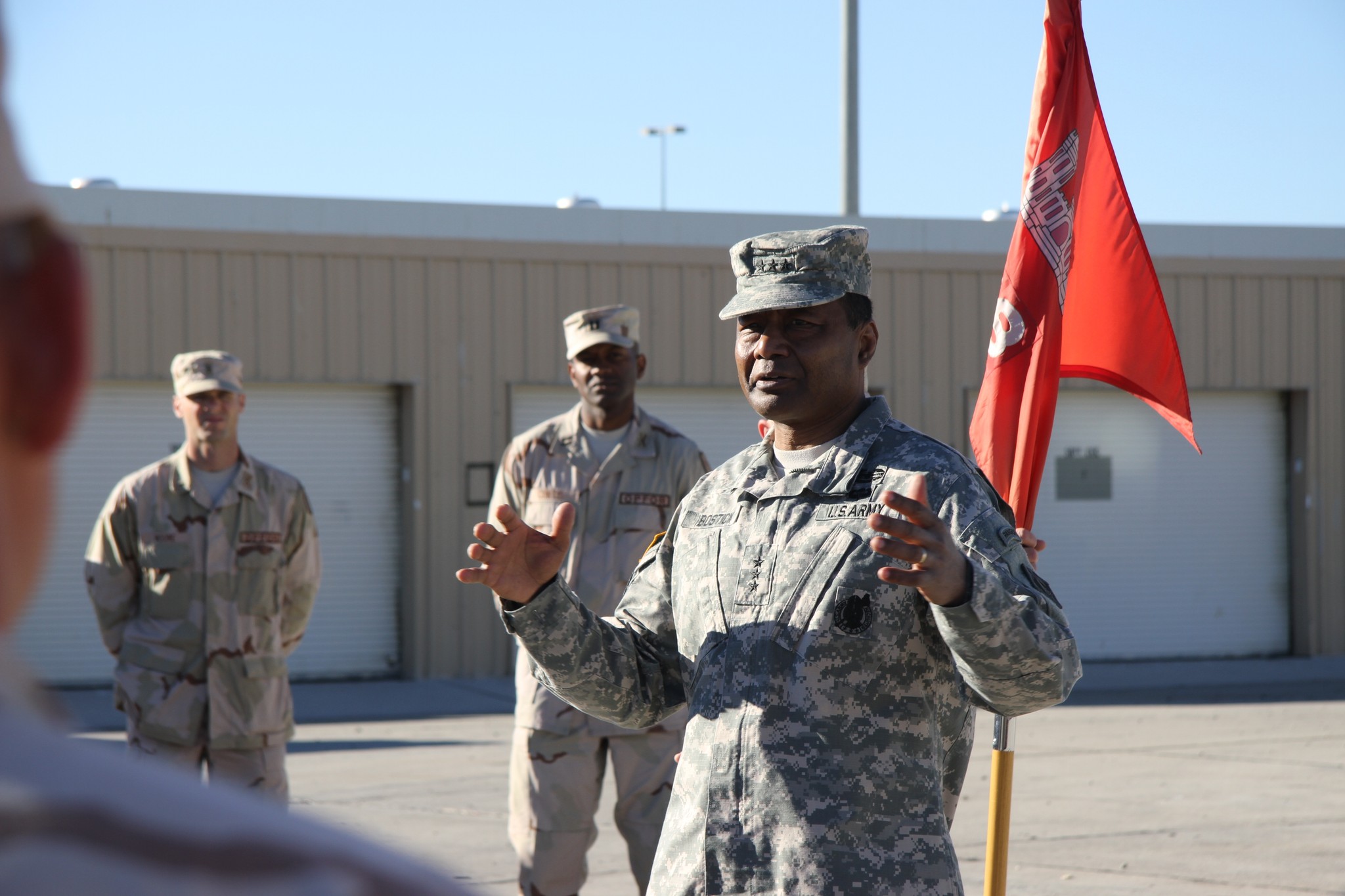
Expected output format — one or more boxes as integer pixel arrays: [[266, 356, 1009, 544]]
[[640, 125, 685, 210]]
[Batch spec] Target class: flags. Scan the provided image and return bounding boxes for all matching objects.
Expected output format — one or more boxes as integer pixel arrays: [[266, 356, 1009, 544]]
[[969, 0, 1204, 532]]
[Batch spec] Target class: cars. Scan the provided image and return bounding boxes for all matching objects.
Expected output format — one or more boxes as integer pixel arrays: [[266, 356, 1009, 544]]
[[982, 205, 1021, 223], [557, 196, 600, 209], [71, 176, 118, 191]]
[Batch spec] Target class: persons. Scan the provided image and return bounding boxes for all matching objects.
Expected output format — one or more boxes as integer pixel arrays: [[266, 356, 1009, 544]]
[[490, 296, 712, 896], [1014, 528, 1046, 571], [0, 19, 479, 896], [77, 350, 319, 812], [453, 221, 1084, 896]]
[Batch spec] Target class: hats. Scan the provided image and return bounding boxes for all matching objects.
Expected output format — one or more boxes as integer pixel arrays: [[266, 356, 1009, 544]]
[[169, 350, 242, 397], [718, 224, 871, 320], [562, 303, 640, 361]]
